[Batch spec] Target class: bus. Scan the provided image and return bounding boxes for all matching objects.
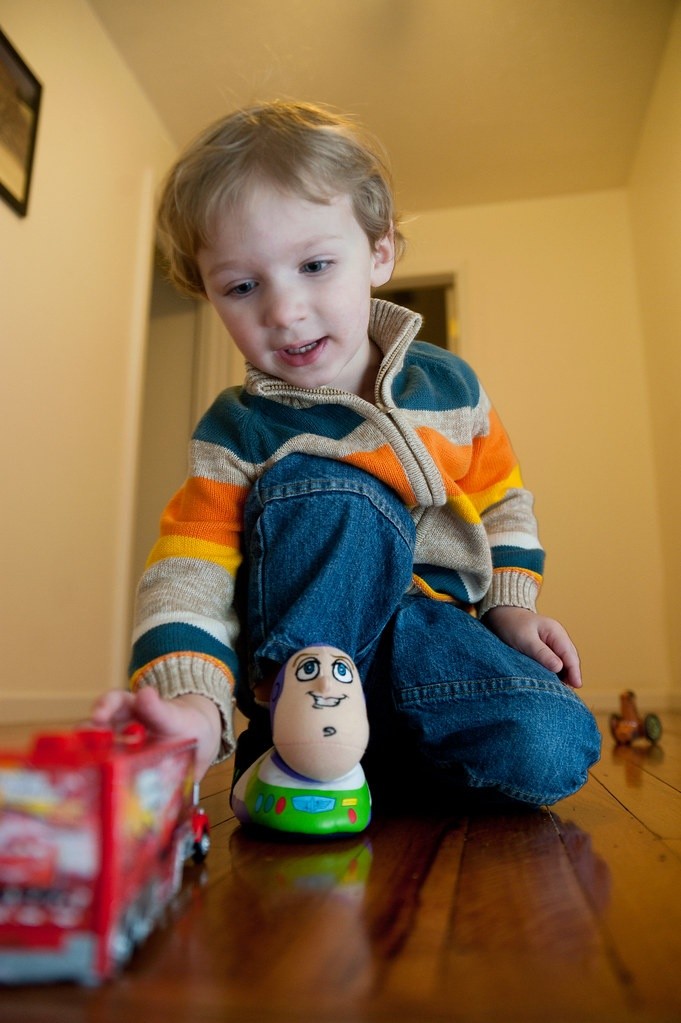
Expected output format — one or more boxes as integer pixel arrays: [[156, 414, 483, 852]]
[[0, 717, 217, 993]]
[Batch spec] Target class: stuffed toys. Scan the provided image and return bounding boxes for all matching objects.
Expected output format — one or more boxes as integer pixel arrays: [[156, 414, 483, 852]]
[[231, 644, 371, 834]]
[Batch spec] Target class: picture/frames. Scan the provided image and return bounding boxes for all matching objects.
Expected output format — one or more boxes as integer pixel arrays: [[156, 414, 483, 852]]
[[0, 29, 44, 219]]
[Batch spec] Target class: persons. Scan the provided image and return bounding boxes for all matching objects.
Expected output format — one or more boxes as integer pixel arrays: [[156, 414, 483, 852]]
[[90, 101, 601, 808]]
